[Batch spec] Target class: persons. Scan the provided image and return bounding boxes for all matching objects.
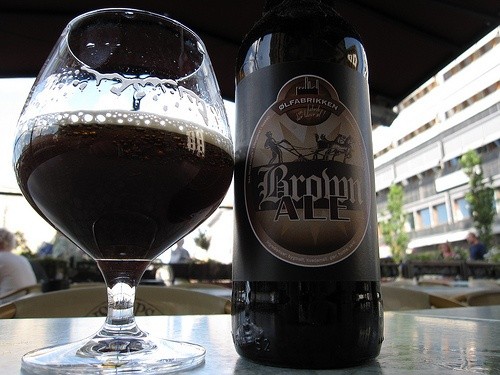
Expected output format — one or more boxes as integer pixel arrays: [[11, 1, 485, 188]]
[[0, 230, 38, 306], [438, 232, 489, 261]]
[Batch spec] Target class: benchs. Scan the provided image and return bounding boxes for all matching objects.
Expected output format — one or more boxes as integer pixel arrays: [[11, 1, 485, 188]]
[[28, 258, 67, 283], [169, 264, 232, 285], [401, 261, 466, 286], [463, 263, 500, 282], [74, 263, 157, 284]]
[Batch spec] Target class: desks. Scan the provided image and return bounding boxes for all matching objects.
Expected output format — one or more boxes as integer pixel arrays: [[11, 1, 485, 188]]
[[0, 306, 500, 375]]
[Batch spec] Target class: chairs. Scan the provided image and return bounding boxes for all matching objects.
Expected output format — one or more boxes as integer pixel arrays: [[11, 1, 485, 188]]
[[453, 289, 500, 306], [380, 286, 466, 310], [0, 284, 231, 319]]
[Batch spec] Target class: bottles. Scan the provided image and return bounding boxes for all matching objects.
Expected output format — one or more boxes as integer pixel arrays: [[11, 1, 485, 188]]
[[231, 1, 385, 371]]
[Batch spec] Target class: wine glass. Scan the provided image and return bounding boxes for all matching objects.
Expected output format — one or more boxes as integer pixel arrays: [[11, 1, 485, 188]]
[[12, 6, 235, 375]]
